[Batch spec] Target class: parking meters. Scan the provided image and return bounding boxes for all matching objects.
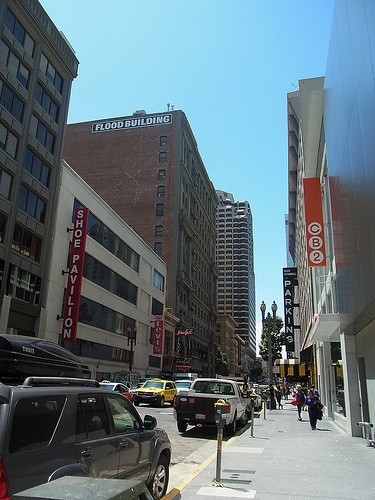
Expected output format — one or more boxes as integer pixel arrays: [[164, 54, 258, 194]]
[[262, 391, 267, 420], [249, 392, 258, 436], [213, 399, 229, 484]]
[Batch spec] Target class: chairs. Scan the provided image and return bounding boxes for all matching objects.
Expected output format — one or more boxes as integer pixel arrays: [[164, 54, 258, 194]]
[[224, 385, 231, 391], [203, 385, 211, 392]]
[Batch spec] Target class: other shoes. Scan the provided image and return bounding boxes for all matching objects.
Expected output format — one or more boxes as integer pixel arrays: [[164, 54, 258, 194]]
[[312, 424, 316, 430], [298, 418, 301, 421], [278, 405, 283, 409]]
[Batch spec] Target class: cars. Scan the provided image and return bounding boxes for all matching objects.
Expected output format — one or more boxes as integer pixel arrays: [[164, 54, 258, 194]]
[[99, 380, 135, 403]]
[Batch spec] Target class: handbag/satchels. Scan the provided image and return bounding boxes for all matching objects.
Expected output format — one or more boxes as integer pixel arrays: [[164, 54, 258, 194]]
[[291, 394, 300, 406]]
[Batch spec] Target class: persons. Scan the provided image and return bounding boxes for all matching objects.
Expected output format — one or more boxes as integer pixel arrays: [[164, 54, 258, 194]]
[[292, 384, 305, 420], [305, 389, 320, 430], [300, 383, 320, 411], [241, 383, 250, 397], [266, 384, 274, 410], [251, 384, 254, 388], [275, 384, 292, 409]]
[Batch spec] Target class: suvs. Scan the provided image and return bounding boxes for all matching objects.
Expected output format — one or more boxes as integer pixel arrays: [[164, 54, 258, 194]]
[[0, 334, 173, 500], [173, 378, 250, 435], [129, 378, 192, 409]]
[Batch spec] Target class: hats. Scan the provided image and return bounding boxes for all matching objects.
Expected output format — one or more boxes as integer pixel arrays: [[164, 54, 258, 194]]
[[297, 385, 301, 390]]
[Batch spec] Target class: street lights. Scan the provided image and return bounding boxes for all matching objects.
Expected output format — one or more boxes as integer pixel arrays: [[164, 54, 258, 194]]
[[127, 327, 138, 382], [260, 301, 277, 409]]
